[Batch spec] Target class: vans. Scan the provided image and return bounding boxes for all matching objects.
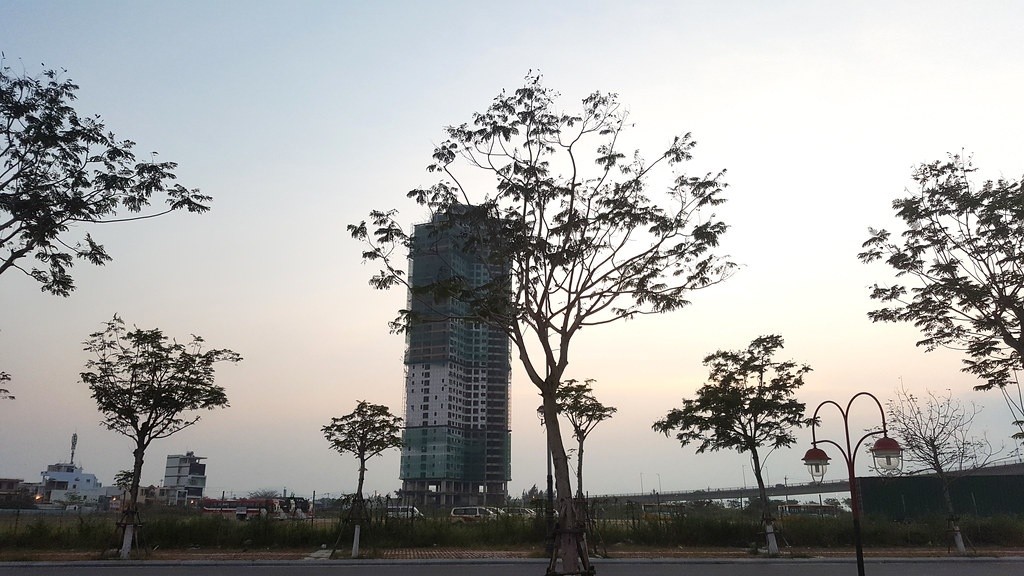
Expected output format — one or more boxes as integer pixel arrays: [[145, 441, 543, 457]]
[[448, 506, 498, 522], [485, 506, 559, 521], [385, 505, 425, 521]]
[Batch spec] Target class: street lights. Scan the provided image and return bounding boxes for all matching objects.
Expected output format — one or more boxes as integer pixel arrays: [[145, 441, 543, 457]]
[[800, 392, 903, 576]]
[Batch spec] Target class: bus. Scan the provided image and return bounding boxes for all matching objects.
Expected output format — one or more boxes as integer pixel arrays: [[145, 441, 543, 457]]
[[203, 495, 312, 521], [778, 505, 834, 519]]
